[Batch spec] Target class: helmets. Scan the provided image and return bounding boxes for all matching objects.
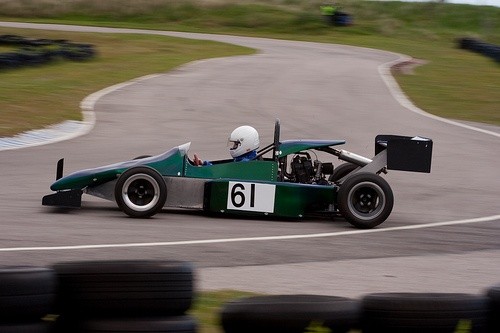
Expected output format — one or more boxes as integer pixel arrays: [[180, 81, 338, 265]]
[[229, 125, 260, 158]]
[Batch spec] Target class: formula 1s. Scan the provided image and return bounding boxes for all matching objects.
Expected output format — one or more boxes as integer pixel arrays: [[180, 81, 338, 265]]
[[41, 119, 433, 227]]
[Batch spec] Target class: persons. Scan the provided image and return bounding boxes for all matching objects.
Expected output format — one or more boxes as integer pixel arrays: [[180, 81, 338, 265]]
[[193, 125, 259, 167]]
